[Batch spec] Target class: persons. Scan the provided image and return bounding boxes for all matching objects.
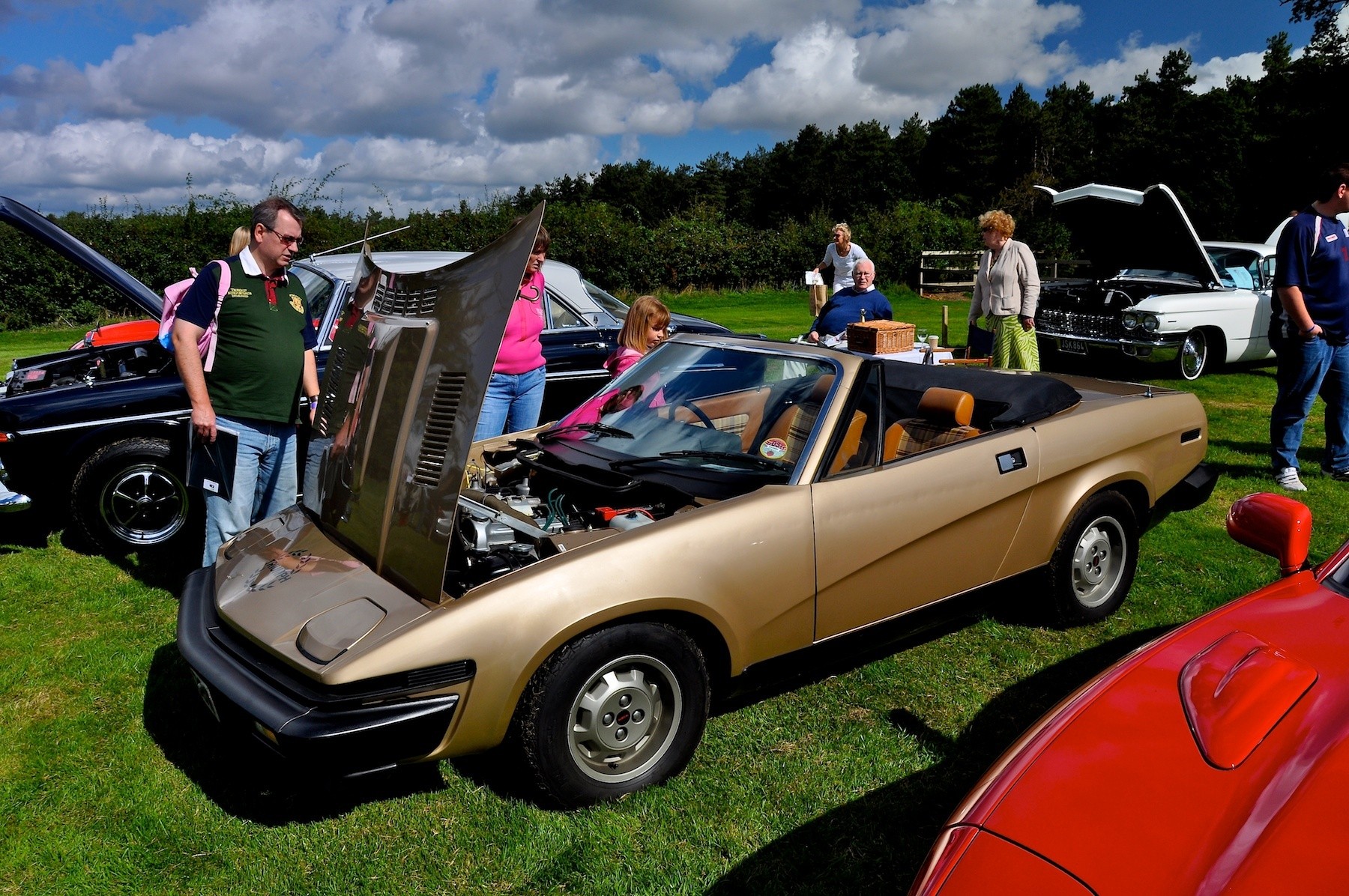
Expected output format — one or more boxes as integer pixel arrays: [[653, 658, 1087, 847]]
[[813, 223, 868, 295], [172, 196, 321, 566], [1268, 169, 1349, 491], [782, 257, 893, 379], [604, 295, 672, 413], [553, 385, 644, 441], [473, 217, 550, 442], [246, 506, 362, 572], [230, 226, 250, 256], [303, 266, 380, 515], [968, 210, 1041, 371]]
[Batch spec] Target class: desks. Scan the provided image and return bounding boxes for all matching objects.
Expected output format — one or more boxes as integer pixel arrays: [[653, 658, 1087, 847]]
[[792, 339, 956, 365]]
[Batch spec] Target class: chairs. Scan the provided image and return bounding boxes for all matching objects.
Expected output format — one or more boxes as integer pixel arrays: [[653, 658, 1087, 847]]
[[918, 319, 996, 367], [778, 375, 868, 476], [881, 387, 982, 462]]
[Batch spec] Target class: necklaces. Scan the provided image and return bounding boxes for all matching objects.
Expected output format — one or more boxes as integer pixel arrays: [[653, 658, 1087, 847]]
[[990, 246, 1004, 267]]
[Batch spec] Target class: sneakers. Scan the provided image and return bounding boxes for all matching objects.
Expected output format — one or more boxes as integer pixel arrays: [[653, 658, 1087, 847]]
[[1275, 467, 1308, 491], [1321, 466, 1349, 482]]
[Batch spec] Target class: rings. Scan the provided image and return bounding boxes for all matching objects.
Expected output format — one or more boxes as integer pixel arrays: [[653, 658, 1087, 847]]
[[1029, 323, 1031, 325]]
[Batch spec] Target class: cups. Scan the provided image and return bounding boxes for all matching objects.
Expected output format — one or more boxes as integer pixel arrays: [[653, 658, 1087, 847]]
[[928, 335, 939, 351]]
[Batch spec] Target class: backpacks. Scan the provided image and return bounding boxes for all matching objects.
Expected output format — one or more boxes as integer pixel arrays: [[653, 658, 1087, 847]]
[[159, 260, 231, 372]]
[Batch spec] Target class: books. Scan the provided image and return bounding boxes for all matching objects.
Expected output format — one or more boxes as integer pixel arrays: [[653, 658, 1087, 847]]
[[186, 421, 240, 501]]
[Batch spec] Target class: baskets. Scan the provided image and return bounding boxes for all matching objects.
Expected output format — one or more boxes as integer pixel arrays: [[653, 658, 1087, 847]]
[[846, 318, 916, 354]]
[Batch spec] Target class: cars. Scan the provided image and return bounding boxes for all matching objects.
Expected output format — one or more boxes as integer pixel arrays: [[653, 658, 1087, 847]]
[[0, 197, 767, 564], [909, 492, 1349, 896], [179, 198, 1220, 809], [1032, 184, 1349, 380]]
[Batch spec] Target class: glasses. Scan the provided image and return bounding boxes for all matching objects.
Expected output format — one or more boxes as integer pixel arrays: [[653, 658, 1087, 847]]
[[982, 225, 997, 232], [263, 223, 303, 246]]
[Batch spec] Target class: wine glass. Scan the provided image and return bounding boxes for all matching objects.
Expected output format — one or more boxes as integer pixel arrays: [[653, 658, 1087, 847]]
[[917, 328, 928, 349]]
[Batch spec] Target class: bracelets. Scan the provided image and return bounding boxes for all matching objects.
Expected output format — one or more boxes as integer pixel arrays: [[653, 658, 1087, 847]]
[[310, 403, 317, 409], [1300, 326, 1315, 333], [309, 395, 319, 403]]
